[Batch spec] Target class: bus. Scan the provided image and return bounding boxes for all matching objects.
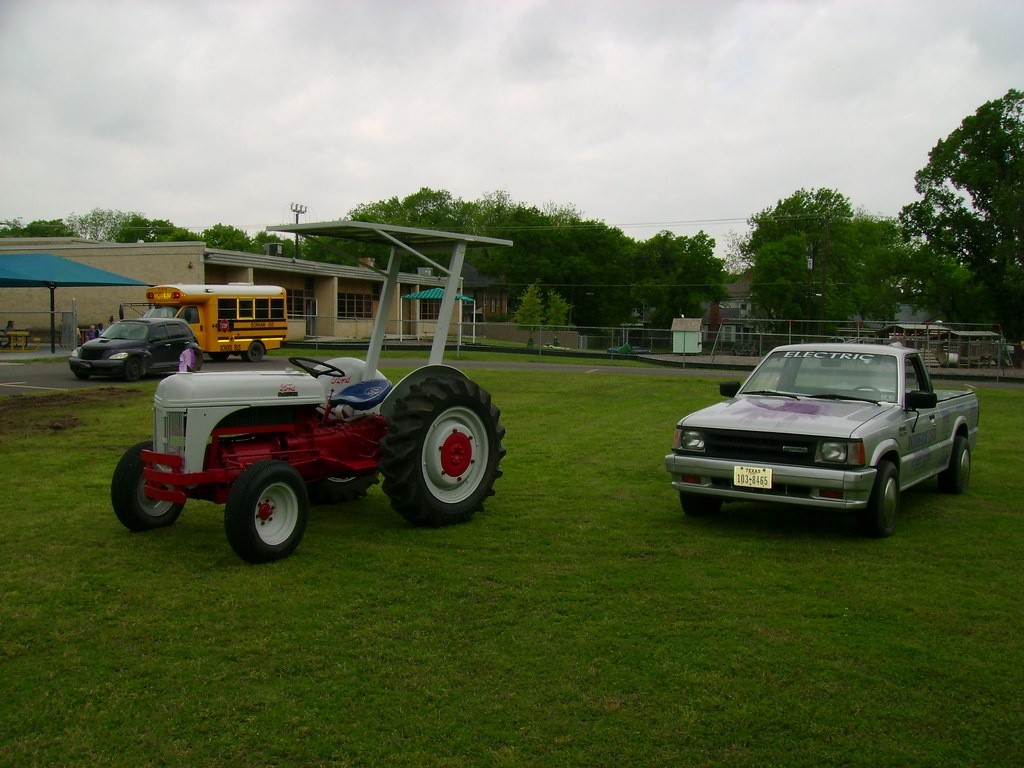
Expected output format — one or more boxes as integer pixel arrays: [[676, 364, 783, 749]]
[[118, 282, 288, 363]]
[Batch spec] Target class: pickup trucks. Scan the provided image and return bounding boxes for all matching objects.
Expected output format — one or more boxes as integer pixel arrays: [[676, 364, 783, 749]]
[[664, 341, 980, 539]]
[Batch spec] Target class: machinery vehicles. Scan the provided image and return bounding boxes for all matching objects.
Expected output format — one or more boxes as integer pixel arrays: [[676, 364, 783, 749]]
[[110, 220, 515, 565]]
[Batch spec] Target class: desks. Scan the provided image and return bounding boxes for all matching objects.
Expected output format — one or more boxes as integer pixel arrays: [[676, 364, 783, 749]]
[[7, 331, 30, 351]]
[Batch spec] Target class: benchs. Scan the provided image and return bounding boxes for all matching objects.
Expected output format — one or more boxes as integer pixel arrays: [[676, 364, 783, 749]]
[[33, 337, 41, 350]]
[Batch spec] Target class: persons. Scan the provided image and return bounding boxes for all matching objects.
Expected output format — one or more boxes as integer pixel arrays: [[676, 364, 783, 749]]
[[85, 323, 104, 343], [3, 321, 14, 348]]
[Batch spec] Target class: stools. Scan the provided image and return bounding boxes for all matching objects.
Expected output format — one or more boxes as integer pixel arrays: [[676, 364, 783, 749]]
[[327, 378, 393, 411]]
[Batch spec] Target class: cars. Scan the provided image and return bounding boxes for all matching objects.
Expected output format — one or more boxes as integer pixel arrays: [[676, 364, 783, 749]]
[[68, 318, 191, 382]]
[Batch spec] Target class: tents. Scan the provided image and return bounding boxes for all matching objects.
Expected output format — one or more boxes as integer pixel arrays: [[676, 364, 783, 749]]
[[0, 252, 158, 353], [400, 288, 475, 346]]
